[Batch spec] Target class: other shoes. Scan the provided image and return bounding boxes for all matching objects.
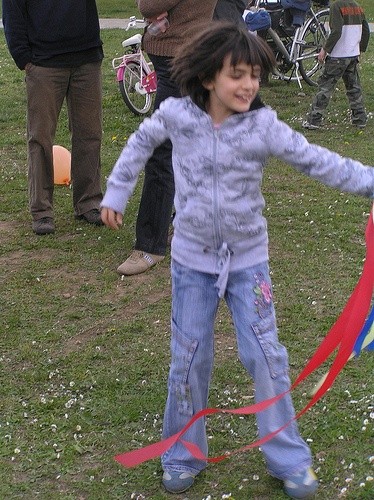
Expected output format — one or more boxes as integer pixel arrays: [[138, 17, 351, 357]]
[[358, 118, 367, 127], [303, 120, 319, 129]]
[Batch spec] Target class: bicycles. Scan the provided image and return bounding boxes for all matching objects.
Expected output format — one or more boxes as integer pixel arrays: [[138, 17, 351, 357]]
[[111, 16, 160, 116], [245, 1, 333, 92]]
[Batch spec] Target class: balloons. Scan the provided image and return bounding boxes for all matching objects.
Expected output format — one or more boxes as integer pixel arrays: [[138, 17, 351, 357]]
[[53, 146, 71, 185]]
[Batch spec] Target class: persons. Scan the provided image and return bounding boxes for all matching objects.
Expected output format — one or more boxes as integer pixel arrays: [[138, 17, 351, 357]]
[[116, 0, 248, 276], [2, 0, 105, 235], [100, 24, 374, 498], [301, 0, 370, 129]]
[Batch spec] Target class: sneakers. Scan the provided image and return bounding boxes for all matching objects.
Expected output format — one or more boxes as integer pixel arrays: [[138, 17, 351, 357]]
[[118, 250, 164, 275], [74, 209, 104, 224], [162, 471, 195, 493], [32, 217, 55, 234], [284, 467, 317, 498]]
